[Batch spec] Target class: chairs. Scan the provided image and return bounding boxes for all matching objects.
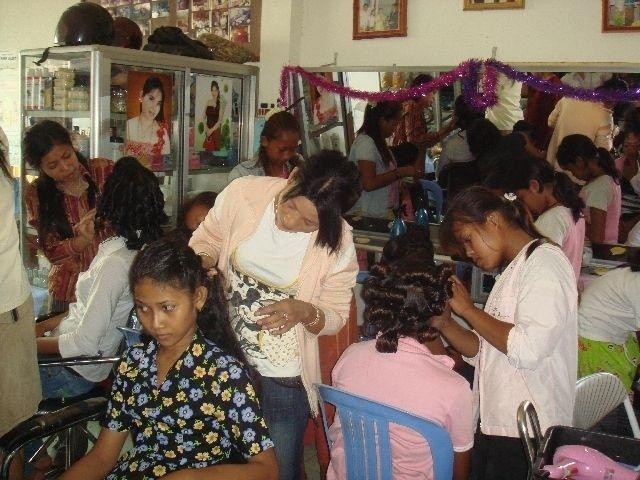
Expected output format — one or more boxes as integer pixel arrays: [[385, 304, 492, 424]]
[[1, 251, 639, 479], [416, 179, 443, 229], [439, 156, 482, 222]]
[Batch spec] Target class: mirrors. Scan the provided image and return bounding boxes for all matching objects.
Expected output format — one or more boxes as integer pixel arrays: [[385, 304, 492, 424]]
[[283, 62, 640, 251]]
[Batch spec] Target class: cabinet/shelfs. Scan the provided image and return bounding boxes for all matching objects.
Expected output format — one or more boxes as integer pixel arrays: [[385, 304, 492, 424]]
[[13, 38, 263, 303], [298, 70, 356, 167]]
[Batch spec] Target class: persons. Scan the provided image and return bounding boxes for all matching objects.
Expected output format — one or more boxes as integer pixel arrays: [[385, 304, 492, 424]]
[[184, 191, 220, 229], [430, 184, 579, 478], [377, 233, 475, 392], [199, 81, 224, 150], [185, 148, 361, 480], [228, 111, 304, 189], [0, 131, 45, 480], [24, 155, 166, 472], [579, 249, 639, 398], [53, 237, 282, 479], [23, 119, 116, 319], [325, 255, 477, 479], [126, 77, 170, 165], [376, 7, 391, 31], [389, 3, 398, 29], [360, 2, 368, 31], [368, 9, 375, 29], [512, 159, 585, 286]]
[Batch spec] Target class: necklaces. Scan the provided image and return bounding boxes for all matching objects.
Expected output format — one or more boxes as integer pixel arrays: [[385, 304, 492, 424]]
[[273, 196, 285, 229]]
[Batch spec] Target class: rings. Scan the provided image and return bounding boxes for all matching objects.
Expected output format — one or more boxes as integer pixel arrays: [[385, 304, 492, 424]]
[[283, 313, 289, 320]]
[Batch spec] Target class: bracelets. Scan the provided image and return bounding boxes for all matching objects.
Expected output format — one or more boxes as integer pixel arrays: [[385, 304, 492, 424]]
[[302, 304, 320, 328]]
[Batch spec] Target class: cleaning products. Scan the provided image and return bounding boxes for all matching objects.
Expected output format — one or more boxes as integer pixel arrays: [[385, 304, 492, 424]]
[[389, 204, 407, 237], [415, 197, 428, 226]]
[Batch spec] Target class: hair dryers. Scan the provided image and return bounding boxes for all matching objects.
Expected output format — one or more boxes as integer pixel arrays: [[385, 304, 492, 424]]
[[543, 444, 640, 480]]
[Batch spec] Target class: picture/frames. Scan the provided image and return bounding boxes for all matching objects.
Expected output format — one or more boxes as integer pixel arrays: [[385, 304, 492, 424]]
[[599, 0, 640, 33], [461, 0, 527, 14], [350, 0, 409, 41]]
